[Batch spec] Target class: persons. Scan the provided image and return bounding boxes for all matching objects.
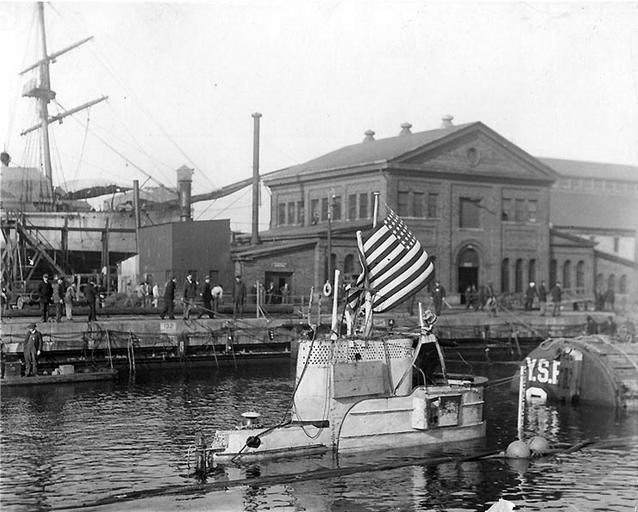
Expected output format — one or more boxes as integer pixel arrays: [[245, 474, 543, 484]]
[[1, 288, 12, 322], [595, 288, 615, 312], [23, 324, 43, 378], [412, 326, 448, 386], [98, 281, 159, 308], [405, 295, 414, 316], [431, 281, 446, 316], [160, 274, 247, 322], [251, 283, 290, 304], [463, 284, 499, 319], [524, 280, 561, 317], [37, 274, 100, 323], [582, 315, 637, 342]]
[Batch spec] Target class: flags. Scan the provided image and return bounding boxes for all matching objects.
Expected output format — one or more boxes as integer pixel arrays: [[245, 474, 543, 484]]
[[343, 202, 436, 316]]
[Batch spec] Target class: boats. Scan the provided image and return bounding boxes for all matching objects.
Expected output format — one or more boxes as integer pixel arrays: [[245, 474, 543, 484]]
[[0, 360, 120, 387], [186, 190, 491, 486]]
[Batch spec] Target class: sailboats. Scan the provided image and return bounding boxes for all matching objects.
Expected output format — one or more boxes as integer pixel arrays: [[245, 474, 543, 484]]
[[0, 2, 195, 280]]
